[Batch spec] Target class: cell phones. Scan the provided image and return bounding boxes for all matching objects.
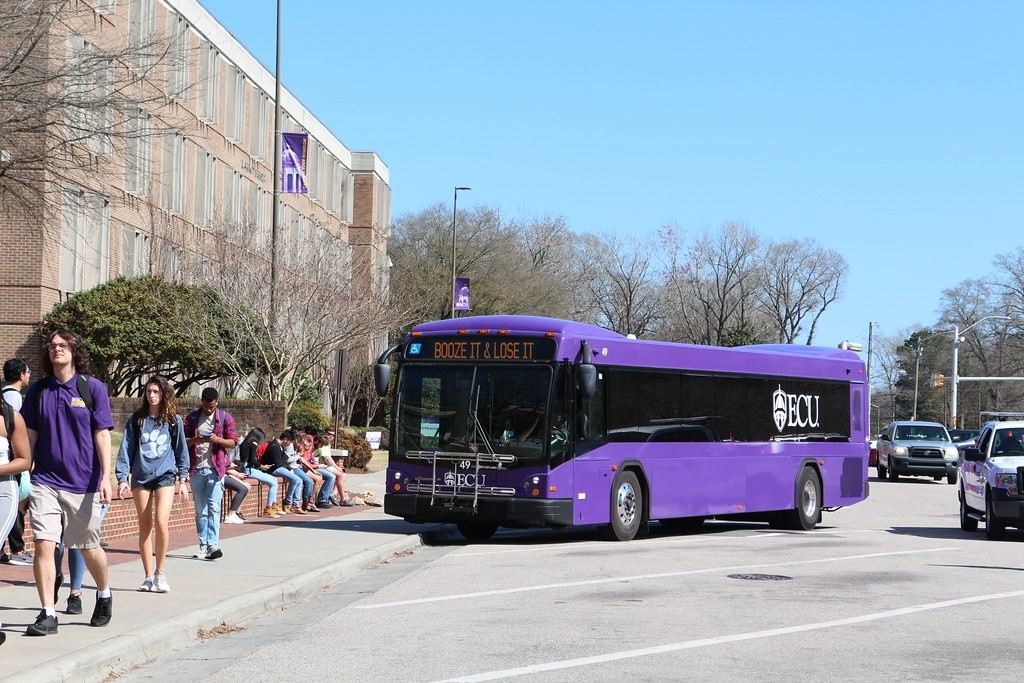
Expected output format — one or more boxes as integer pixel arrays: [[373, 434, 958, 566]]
[[270, 464, 275, 467], [203, 434, 211, 438]]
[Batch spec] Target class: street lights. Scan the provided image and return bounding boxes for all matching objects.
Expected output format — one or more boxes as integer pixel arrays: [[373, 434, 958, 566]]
[[914, 330, 954, 420], [950, 317, 1011, 429], [452, 187, 472, 319]]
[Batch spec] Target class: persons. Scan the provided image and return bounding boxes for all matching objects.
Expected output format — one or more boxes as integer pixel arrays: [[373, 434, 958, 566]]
[[1, 358, 35, 566], [313, 436, 336, 508], [259, 429, 307, 514], [115, 374, 190, 592], [324, 427, 355, 506], [281, 428, 313, 511], [18, 329, 115, 635], [184, 387, 238, 561], [297, 434, 322, 511], [224, 462, 252, 524], [54, 504, 110, 615], [0, 373, 32, 647], [238, 427, 287, 517]]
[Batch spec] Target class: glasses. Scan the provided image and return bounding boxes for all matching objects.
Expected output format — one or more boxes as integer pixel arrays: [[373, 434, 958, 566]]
[[23, 369, 32, 375], [48, 344, 73, 350]]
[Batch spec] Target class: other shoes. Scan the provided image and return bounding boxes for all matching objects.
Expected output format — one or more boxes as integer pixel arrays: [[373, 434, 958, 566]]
[[193, 551, 207, 559], [55, 572, 64, 605], [205, 549, 222, 559]]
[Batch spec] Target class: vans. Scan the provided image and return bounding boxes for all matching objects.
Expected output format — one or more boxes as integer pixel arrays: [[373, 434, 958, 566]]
[[595, 424, 715, 443]]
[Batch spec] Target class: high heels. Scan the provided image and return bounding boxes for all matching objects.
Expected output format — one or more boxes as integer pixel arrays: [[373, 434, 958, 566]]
[[301, 502, 320, 512]]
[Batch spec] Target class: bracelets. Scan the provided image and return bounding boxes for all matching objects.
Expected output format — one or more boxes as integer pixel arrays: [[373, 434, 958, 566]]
[[180, 480, 185, 484]]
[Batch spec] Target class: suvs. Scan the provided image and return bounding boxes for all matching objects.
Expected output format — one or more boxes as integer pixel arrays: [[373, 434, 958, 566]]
[[958, 420, 1024, 540], [877, 420, 960, 485]]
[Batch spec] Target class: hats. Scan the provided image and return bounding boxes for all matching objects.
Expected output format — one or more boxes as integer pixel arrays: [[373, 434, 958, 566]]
[[306, 434, 314, 442]]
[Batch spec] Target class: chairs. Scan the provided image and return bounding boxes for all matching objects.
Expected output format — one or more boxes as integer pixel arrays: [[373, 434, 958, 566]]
[[996, 436, 1024, 452]]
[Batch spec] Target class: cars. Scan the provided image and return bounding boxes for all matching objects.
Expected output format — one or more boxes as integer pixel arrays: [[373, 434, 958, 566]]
[[936, 429, 981, 455]]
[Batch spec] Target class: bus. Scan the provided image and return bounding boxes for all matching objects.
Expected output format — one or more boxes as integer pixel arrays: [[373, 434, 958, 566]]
[[374, 314, 869, 542]]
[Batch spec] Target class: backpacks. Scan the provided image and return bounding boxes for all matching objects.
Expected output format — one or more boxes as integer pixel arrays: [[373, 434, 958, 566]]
[[256, 440, 280, 462], [229, 434, 245, 467]]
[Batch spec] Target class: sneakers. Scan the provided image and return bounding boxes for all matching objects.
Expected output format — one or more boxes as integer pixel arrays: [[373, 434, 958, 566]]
[[0, 552, 10, 564], [272, 505, 287, 515], [66, 592, 82, 614], [90, 588, 113, 627], [153, 573, 170, 592], [315, 493, 353, 508], [8, 550, 35, 566], [26, 609, 59, 636], [223, 512, 247, 524], [283, 501, 307, 514], [139, 575, 154, 593], [263, 507, 281, 518]]
